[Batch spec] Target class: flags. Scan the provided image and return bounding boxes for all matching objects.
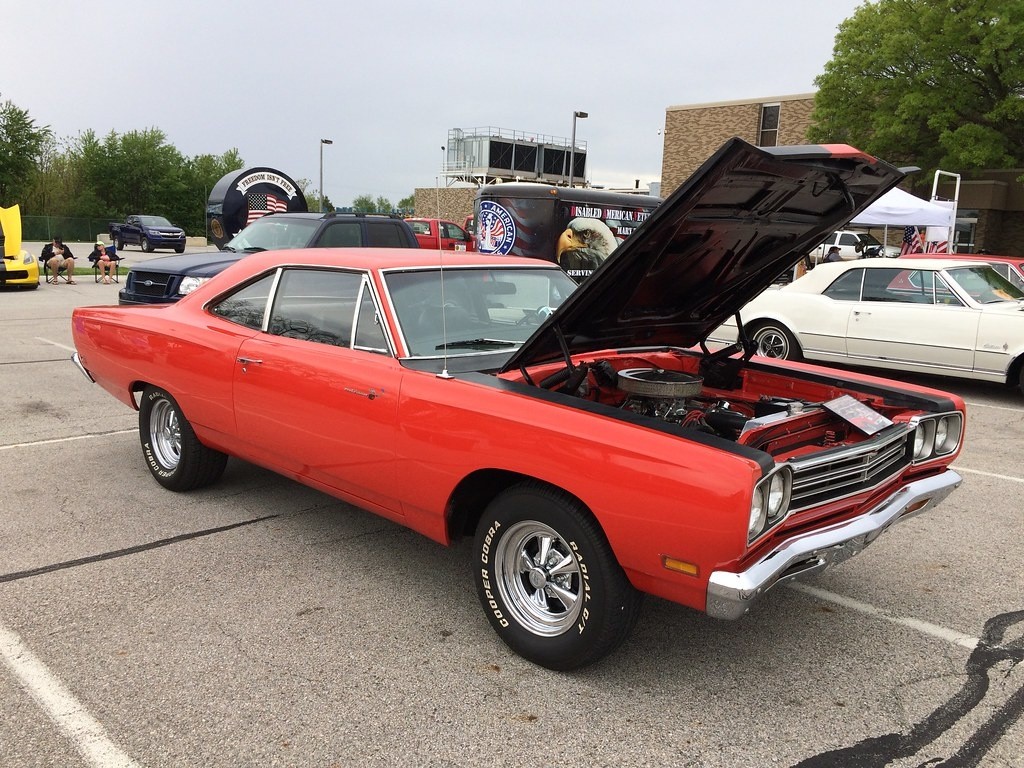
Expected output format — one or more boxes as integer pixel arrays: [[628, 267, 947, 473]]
[[900, 226, 921, 255], [929, 241, 947, 253]]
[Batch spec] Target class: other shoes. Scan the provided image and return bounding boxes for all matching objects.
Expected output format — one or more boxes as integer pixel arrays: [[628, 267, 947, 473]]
[[103, 280, 107, 283], [66, 281, 77, 284], [107, 280, 110, 283], [53, 281, 59, 284]]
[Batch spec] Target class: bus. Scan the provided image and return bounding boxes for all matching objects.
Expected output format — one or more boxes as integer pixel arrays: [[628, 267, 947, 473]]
[[475, 183, 666, 282]]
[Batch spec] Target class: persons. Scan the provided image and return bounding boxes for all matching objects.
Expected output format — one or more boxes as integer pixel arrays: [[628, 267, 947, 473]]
[[824, 247, 843, 262], [88, 241, 120, 284], [40, 235, 76, 285]]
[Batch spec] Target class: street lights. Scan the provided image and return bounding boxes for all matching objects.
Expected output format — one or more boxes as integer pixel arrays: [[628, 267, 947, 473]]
[[319, 138, 333, 212], [568, 111, 589, 188]]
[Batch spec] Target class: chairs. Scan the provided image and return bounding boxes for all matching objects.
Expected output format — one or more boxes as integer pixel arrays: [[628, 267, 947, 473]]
[[92, 245, 125, 284], [39, 254, 78, 284]]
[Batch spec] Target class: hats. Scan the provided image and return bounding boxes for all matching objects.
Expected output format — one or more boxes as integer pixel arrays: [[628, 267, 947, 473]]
[[829, 246, 841, 251], [95, 241, 104, 245]]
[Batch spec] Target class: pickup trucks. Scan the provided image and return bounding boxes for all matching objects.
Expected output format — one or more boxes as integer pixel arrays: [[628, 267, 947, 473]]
[[108, 214, 187, 254]]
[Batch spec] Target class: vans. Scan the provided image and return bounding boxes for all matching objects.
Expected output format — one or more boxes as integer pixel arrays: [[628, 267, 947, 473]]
[[462, 216, 475, 236]]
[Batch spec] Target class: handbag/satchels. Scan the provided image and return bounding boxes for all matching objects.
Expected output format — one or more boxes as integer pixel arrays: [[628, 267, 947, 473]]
[[823, 252, 835, 263]]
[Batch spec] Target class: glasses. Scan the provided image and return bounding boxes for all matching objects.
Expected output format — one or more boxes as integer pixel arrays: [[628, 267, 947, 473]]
[[55, 240, 62, 243]]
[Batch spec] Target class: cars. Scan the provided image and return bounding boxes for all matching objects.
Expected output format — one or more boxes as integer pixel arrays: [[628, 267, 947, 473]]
[[804, 229, 902, 273], [0, 204, 41, 290], [69, 135, 968, 673], [703, 252, 1024, 399]]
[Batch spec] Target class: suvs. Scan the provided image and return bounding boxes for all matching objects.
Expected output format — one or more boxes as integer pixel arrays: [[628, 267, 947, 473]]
[[117, 209, 423, 304], [402, 217, 477, 251]]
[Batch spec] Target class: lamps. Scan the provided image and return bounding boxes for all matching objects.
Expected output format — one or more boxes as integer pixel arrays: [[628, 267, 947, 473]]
[[658, 126, 668, 136]]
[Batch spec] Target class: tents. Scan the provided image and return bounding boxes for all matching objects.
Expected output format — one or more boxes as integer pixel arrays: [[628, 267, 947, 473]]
[[814, 187, 955, 266]]
